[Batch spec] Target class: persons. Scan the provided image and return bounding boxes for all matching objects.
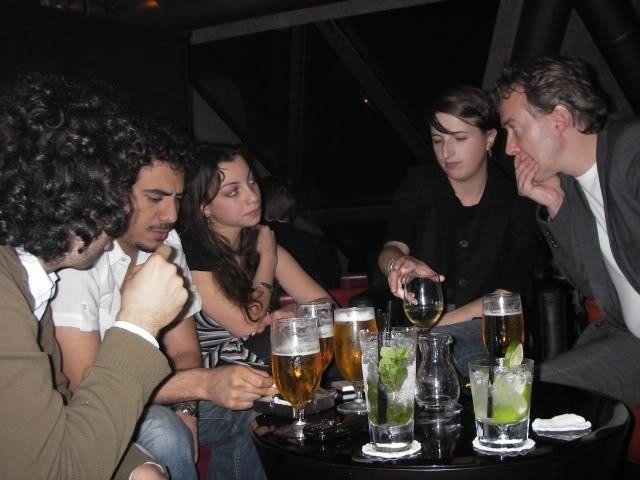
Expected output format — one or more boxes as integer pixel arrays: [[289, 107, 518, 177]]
[[1, 69, 188, 479], [176, 138, 345, 385], [49, 119, 277, 479], [497, 51, 639, 412], [255, 174, 342, 293], [349, 86, 552, 389]]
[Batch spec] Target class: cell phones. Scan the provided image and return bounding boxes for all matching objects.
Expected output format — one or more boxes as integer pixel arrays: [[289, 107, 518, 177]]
[[337, 390, 358, 402], [331, 380, 356, 392]]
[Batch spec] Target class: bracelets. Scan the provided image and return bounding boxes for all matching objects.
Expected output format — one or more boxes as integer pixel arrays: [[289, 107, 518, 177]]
[[387, 257, 404, 272], [171, 400, 200, 420]]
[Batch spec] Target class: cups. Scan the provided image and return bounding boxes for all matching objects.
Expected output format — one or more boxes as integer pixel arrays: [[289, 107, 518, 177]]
[[468, 357, 534, 448], [359, 326, 419, 453], [481, 294, 526, 357], [403, 273, 444, 334]]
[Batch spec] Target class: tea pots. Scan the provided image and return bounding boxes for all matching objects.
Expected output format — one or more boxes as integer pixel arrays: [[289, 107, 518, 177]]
[[416, 333, 462, 411]]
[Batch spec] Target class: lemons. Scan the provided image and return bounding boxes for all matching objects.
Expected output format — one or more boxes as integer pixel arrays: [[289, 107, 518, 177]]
[[493, 339, 531, 424]]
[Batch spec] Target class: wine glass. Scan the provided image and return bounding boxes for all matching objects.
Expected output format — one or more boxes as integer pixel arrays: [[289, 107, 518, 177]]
[[271, 316, 323, 437], [333, 306, 379, 414], [296, 301, 335, 399]]
